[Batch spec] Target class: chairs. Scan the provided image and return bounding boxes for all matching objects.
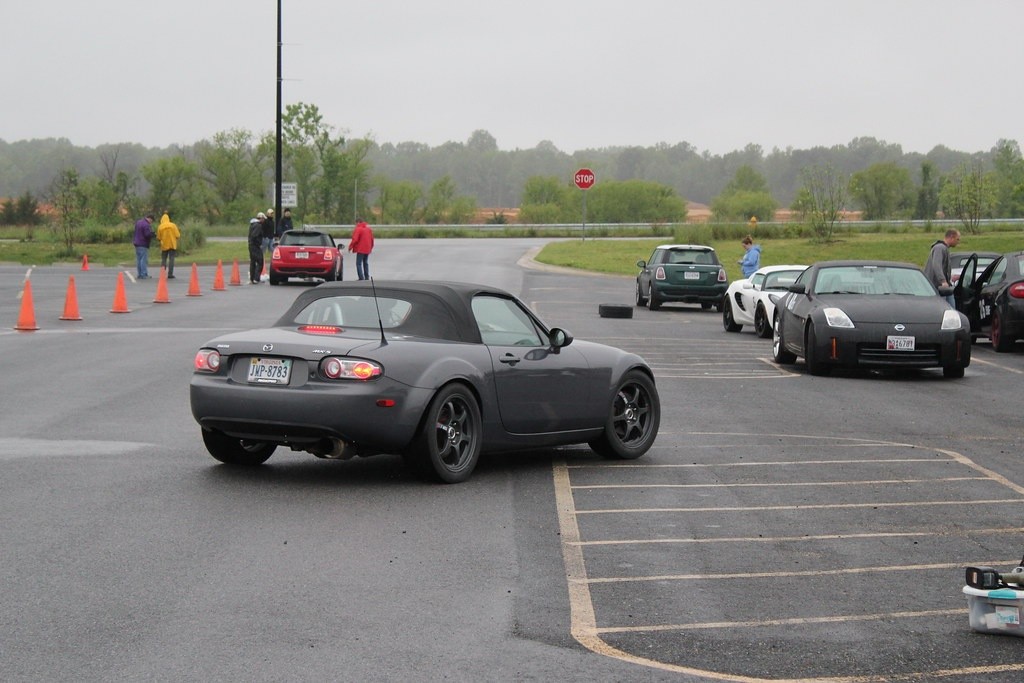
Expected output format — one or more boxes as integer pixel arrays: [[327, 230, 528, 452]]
[[669, 253, 677, 262], [695, 253, 706, 263], [820, 273, 843, 290]]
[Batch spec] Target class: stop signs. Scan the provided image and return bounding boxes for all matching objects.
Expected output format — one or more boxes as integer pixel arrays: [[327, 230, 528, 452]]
[[574, 168, 595, 189]]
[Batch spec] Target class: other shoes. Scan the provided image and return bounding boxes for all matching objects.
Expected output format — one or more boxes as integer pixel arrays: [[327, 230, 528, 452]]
[[168, 275, 175, 278], [139, 275, 152, 280]]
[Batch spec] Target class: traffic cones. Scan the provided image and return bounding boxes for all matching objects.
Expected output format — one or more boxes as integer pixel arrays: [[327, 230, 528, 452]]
[[228, 258, 242, 286], [13, 279, 41, 330], [80, 255, 90, 271], [109, 272, 132, 313], [185, 262, 203, 296], [58, 275, 83, 321], [211, 259, 226, 291], [153, 267, 171, 303]]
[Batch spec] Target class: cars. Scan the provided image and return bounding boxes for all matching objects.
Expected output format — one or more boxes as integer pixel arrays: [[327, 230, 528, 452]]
[[954, 252, 1024, 353], [635, 243, 729, 312], [187, 280, 661, 485], [948, 252, 1001, 286], [772, 259, 970, 379], [722, 265, 809, 338], [269, 229, 345, 285]]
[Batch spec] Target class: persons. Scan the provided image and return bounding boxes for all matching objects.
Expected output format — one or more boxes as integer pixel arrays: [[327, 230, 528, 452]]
[[133, 214, 156, 280], [260, 209, 276, 255], [348, 217, 374, 280], [247, 212, 266, 284], [737, 235, 762, 279], [277, 207, 294, 240], [156, 213, 181, 278], [923, 228, 961, 310]]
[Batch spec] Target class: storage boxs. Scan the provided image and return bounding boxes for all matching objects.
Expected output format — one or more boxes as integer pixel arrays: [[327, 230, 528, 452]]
[[961, 584, 1024, 637]]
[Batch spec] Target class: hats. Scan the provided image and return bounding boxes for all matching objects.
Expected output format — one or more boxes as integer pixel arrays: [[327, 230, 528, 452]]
[[257, 213, 267, 219], [284, 208, 290, 212], [147, 215, 155, 221]]
